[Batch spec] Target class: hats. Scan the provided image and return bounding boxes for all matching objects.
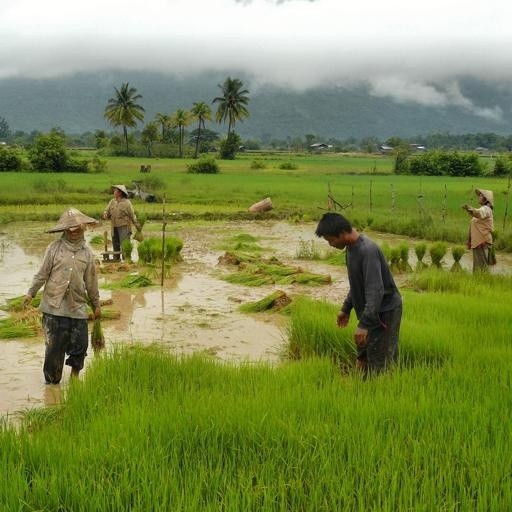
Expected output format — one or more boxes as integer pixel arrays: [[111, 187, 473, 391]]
[[111, 184, 128, 194], [45, 208, 99, 234], [475, 189, 494, 210]]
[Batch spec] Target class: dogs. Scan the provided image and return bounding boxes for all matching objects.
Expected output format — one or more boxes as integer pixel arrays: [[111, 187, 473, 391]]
[[139, 163, 152, 173]]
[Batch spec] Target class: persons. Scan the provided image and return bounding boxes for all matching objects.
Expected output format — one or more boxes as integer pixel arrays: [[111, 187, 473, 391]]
[[315, 212, 402, 381], [462, 188, 495, 275], [101, 183, 142, 262], [23, 207, 101, 385]]
